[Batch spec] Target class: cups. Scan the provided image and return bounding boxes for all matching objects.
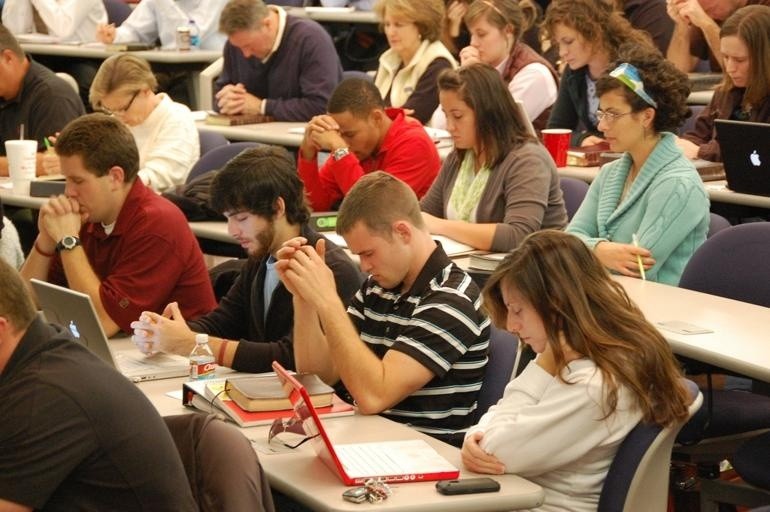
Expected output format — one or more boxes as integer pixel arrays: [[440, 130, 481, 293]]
[[3, 138, 39, 198], [541, 129, 572, 169]]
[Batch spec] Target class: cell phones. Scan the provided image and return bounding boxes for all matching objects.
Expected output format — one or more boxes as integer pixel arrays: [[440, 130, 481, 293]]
[[435, 478, 500, 495]]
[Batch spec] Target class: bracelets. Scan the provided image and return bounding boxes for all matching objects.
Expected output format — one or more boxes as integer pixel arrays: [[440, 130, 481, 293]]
[[217, 340, 229, 367], [34, 240, 56, 256]]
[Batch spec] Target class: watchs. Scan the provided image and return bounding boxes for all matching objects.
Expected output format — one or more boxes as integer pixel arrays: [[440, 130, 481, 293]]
[[332, 147, 350, 162], [54, 235, 81, 257]]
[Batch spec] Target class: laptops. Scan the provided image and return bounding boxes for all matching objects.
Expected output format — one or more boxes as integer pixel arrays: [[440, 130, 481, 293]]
[[30, 277, 188, 382], [714, 119, 770, 197], [272, 361, 460, 486]]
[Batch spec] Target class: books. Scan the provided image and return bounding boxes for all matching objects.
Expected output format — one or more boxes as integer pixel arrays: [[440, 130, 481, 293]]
[[470, 255, 504, 270], [428, 234, 478, 259], [180, 371, 355, 428]]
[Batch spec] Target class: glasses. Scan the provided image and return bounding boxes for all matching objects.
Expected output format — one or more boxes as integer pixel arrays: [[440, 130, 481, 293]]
[[99, 90, 139, 116]]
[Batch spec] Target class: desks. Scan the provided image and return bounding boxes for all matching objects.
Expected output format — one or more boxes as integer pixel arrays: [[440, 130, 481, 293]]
[[0, 4, 600, 220], [0, 172, 504, 294], [681, 68, 770, 209], [110, 332, 546, 511]]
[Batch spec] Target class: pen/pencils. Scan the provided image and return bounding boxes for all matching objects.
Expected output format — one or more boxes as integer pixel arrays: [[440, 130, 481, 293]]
[[632, 233, 646, 281], [44, 137, 53, 155]]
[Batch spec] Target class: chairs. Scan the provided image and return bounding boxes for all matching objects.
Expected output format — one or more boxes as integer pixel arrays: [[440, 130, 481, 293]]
[[597, 209, 770, 511], [479, 324, 522, 412]]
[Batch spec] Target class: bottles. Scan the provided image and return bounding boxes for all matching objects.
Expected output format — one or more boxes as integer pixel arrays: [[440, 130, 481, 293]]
[[188, 333, 215, 382], [176, 26, 190, 51], [188, 19, 200, 52]]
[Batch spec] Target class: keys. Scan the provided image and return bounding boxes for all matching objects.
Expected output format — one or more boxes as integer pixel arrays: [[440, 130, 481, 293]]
[[343, 482, 388, 503]]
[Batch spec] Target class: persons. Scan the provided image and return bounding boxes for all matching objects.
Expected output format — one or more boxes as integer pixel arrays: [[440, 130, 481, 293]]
[[0, 260, 198, 512], [129, 146, 363, 403], [412, 62, 568, 254], [0, 0, 343, 197], [275, 171, 488, 449], [16, 114, 219, 341], [371, 0, 769, 149], [456, 232, 693, 512], [558, 52, 710, 281], [299, 78, 441, 208]]
